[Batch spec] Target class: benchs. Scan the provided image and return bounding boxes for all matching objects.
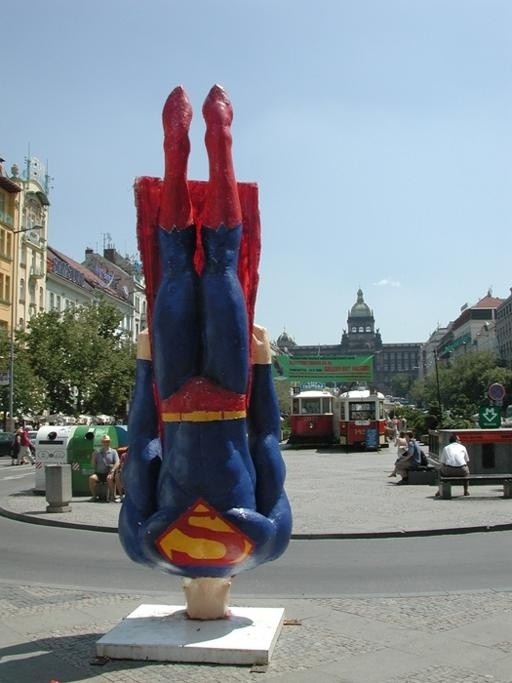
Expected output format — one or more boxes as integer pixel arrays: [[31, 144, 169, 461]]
[[435, 469, 511, 496], [99, 478, 119, 501], [399, 457, 439, 482]]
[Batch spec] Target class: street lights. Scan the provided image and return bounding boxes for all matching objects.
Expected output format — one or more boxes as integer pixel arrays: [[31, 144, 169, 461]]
[[8, 223, 43, 433]]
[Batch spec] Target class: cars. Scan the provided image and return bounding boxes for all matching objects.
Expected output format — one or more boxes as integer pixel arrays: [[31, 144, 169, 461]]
[[1, 429, 38, 455], [391, 394, 512, 427]]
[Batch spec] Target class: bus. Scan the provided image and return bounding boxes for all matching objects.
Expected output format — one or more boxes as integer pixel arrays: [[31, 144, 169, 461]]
[[336, 388, 388, 446], [285, 384, 342, 444]]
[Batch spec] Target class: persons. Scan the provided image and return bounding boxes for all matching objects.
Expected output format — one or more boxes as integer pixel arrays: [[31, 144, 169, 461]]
[[115, 448, 130, 502], [11, 428, 23, 465], [16, 426, 36, 465], [118, 85, 293, 622], [89, 434, 120, 502], [435, 435, 471, 496], [384, 415, 421, 485]]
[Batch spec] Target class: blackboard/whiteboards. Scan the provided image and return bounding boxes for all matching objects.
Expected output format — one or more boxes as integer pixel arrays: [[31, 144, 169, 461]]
[[365, 427, 378, 450]]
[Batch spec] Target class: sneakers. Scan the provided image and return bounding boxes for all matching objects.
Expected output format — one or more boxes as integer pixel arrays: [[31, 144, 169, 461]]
[[88, 497, 97, 502], [114, 497, 123, 503]]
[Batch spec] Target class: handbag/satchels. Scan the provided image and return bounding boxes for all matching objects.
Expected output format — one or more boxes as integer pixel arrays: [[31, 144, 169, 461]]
[[421, 450, 429, 466], [10, 447, 18, 459]]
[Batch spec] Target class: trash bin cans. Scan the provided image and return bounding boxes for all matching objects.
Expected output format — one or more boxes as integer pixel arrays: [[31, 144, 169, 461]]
[[33, 425, 128, 497]]
